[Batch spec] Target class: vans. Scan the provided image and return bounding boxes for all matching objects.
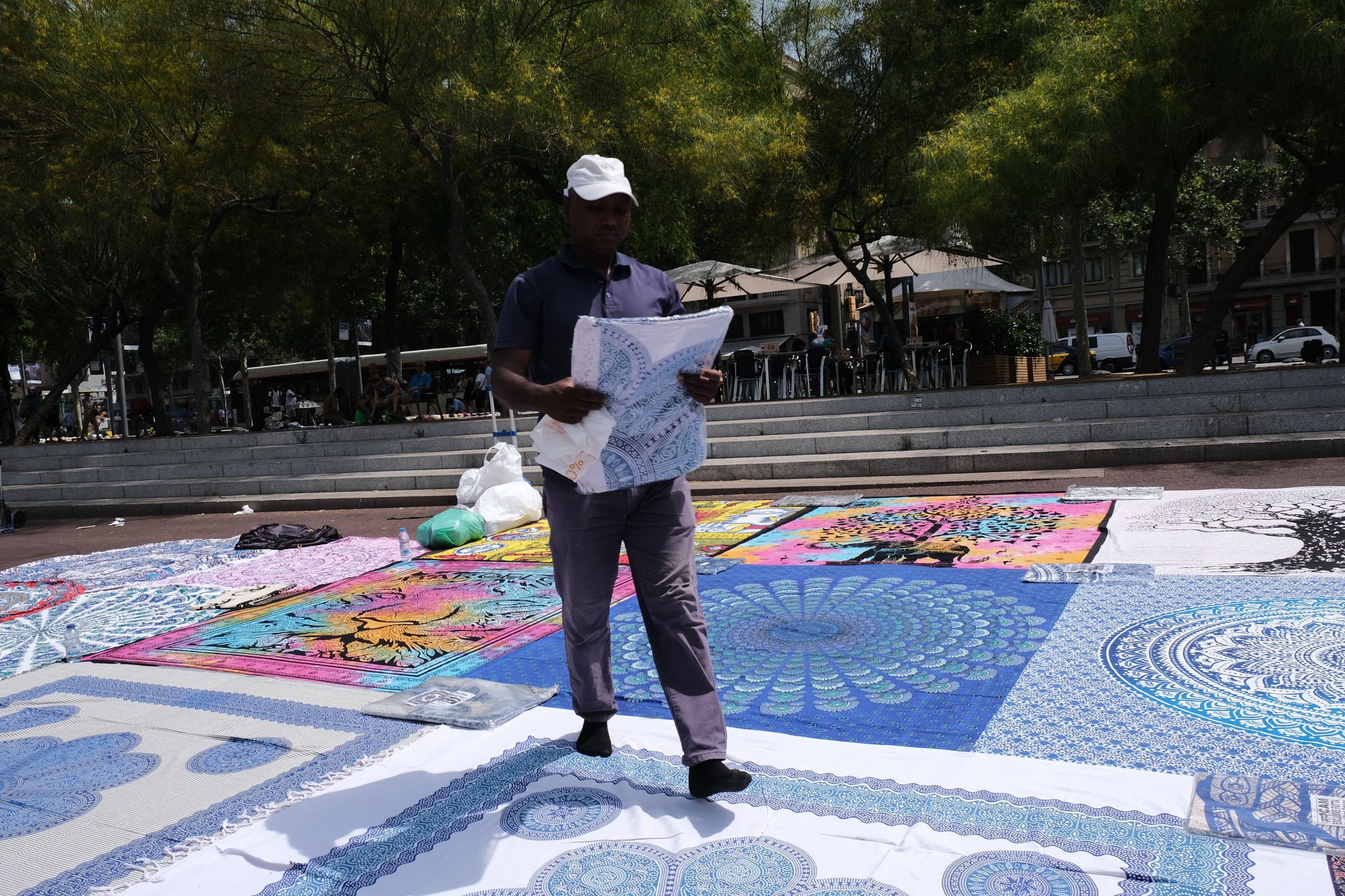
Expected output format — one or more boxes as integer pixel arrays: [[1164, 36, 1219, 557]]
[[1037, 332, 1137, 376]]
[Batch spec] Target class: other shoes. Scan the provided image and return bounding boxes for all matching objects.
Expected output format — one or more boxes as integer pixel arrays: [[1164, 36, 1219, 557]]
[[388, 412, 399, 417]]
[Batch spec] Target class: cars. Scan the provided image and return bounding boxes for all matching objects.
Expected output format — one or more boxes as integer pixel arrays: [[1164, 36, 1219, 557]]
[[1246, 326, 1340, 363], [1159, 334, 1229, 370]]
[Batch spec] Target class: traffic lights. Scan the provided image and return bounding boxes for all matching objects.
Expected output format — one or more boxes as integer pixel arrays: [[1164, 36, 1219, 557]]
[[809, 311, 818, 332]]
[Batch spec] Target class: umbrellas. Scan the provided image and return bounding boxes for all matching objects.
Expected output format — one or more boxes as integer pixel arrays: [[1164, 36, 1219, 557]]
[[1041, 299, 1058, 380], [665, 234, 1037, 369]]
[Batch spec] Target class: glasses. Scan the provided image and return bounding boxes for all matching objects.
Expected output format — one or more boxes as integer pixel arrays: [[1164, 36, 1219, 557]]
[[28, 389, 34, 391], [416, 365, 422, 367]]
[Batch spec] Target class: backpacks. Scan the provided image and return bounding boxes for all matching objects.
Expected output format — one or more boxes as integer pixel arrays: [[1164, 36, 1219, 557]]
[[234, 521, 343, 552]]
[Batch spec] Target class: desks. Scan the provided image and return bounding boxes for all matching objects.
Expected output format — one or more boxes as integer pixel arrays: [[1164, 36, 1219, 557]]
[[291, 400, 323, 429]]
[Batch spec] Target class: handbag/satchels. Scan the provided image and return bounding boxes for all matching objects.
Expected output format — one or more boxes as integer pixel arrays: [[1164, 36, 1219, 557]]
[[94, 410, 99, 416], [355, 409, 368, 425], [475, 387, 482, 396], [385, 409, 393, 423], [416, 440, 543, 551]]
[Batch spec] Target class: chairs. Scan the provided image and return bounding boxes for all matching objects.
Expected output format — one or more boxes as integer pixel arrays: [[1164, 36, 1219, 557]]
[[719, 337, 975, 401], [395, 380, 444, 420]]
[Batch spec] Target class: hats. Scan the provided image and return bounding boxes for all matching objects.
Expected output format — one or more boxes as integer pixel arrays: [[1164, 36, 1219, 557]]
[[30, 386, 36, 389], [83, 392, 92, 397], [563, 153, 640, 207]]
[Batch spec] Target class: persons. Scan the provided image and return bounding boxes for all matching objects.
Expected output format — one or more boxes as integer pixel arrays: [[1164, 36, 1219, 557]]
[[791, 338, 807, 373], [1210, 329, 1232, 370], [213, 407, 233, 426], [810, 331, 823, 350], [87, 403, 109, 431], [866, 340, 878, 353], [446, 398, 466, 417], [1296, 319, 1305, 327], [387, 360, 432, 418], [356, 362, 493, 423], [268, 383, 302, 429], [185, 406, 198, 433], [126, 397, 156, 438], [1247, 321, 1257, 348], [822, 329, 834, 348], [487, 153, 753, 799], [81, 392, 104, 440], [323, 387, 350, 426], [11, 386, 79, 444]]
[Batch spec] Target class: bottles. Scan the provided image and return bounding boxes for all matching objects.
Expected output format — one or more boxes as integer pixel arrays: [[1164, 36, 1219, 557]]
[[398, 528, 412, 562], [64, 624, 82, 663]]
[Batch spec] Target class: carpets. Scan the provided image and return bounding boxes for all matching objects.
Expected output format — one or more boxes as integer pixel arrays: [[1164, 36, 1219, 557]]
[[0, 485, 1344, 895]]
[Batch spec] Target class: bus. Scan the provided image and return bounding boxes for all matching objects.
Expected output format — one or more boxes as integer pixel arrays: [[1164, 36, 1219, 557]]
[[229, 343, 489, 428]]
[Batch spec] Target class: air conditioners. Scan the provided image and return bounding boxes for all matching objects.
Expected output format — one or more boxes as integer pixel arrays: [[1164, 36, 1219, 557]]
[[1263, 202, 1282, 218]]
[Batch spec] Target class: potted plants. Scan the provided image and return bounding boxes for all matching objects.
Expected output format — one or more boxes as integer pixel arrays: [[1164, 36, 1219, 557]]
[[960, 301, 1047, 385]]
